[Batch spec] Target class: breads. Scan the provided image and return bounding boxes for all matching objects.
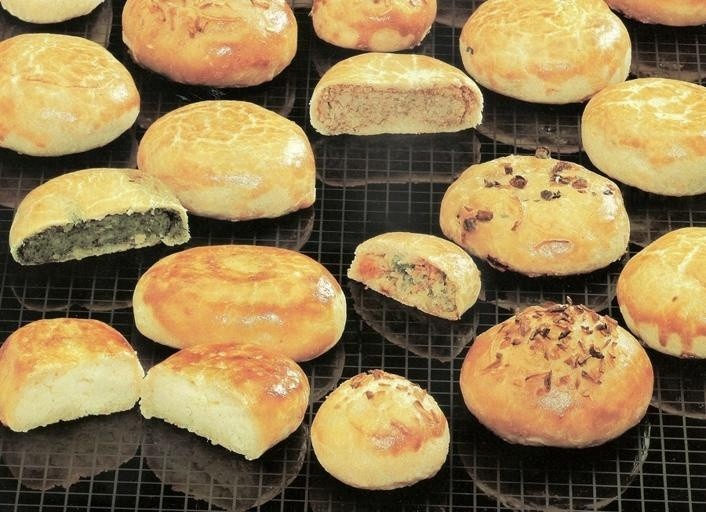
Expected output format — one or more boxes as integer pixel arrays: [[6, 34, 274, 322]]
[[130, 244, 348, 364], [310, 0, 439, 54], [9, 166, 192, 267], [458, 0, 632, 105], [121, 0, 299, 89], [581, 77, 706, 198], [438, 154, 631, 278], [304, 51, 485, 136], [607, 0, 706, 27], [0, 318, 146, 431], [309, 368, 451, 493], [138, 341, 311, 461], [347, 230, 483, 321], [615, 228, 705, 360], [1, 0, 103, 25], [0, 33, 142, 157], [135, 99, 317, 223], [457, 297, 655, 448]]
